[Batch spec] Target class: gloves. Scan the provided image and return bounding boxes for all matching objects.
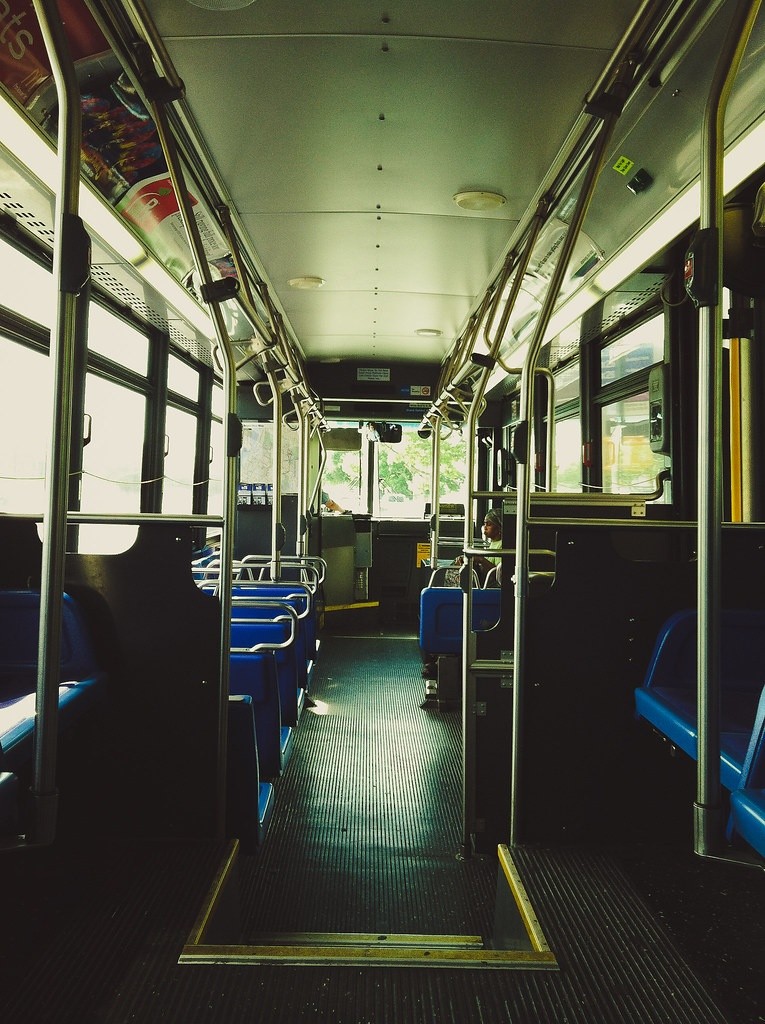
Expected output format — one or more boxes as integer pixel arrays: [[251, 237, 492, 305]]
[[342, 510, 352, 515]]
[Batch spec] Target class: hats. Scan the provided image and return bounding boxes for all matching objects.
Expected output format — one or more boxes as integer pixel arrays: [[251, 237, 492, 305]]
[[487, 508, 502, 527]]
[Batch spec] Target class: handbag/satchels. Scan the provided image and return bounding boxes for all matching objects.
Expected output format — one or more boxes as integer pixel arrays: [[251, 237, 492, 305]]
[[444, 561, 476, 588], [472, 555, 497, 586]]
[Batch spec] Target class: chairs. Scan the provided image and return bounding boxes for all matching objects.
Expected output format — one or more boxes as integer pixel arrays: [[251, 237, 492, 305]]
[[420, 565, 501, 654], [637, 606, 765, 860], [0, 553, 325, 856]]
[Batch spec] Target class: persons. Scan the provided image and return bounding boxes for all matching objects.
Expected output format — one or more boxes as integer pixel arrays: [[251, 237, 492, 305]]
[[421, 509, 502, 678], [321, 490, 348, 514]]
